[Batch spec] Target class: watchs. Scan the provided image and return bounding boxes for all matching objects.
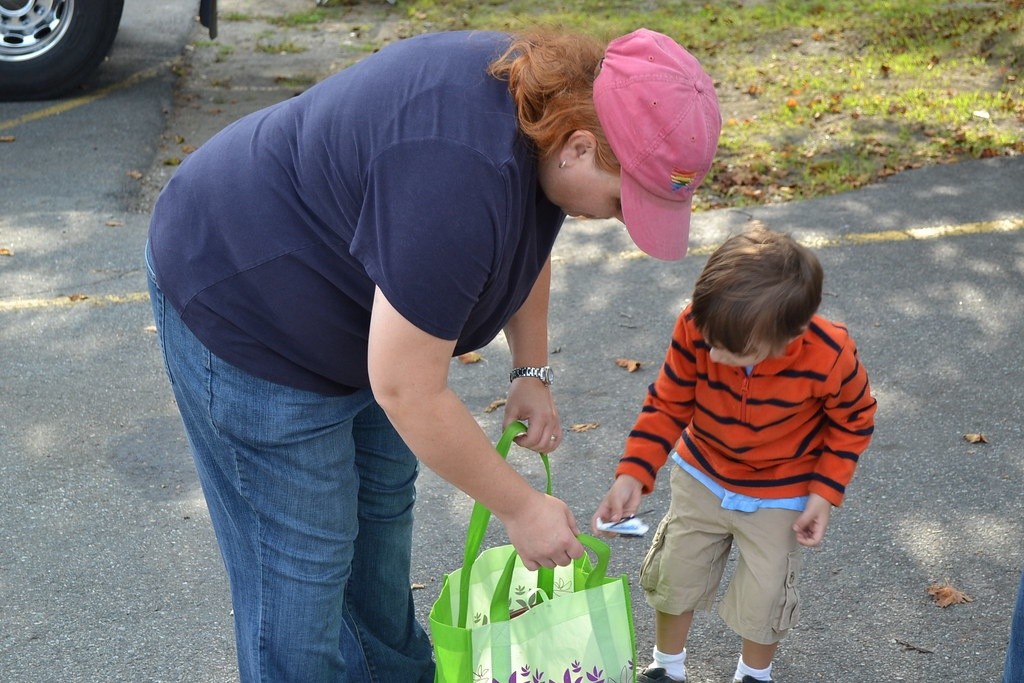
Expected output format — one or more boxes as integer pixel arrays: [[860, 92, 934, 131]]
[[507, 365, 554, 386]]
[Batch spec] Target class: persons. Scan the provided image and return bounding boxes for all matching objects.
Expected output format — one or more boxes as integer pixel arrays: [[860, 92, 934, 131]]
[[590, 220, 877, 683], [144, 22, 721, 682]]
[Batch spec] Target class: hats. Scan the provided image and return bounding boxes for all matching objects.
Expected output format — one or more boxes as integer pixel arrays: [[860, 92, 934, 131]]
[[594, 27, 722, 262]]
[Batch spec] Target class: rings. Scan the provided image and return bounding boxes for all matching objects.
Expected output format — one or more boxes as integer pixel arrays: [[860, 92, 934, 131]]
[[549, 434, 557, 442]]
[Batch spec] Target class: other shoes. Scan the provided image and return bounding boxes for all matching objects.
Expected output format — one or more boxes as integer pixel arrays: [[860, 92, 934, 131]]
[[733, 674, 774, 683], [637, 667, 685, 683]]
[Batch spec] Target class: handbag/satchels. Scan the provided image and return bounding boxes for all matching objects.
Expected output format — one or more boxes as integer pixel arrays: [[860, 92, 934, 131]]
[[428, 419, 636, 683]]
